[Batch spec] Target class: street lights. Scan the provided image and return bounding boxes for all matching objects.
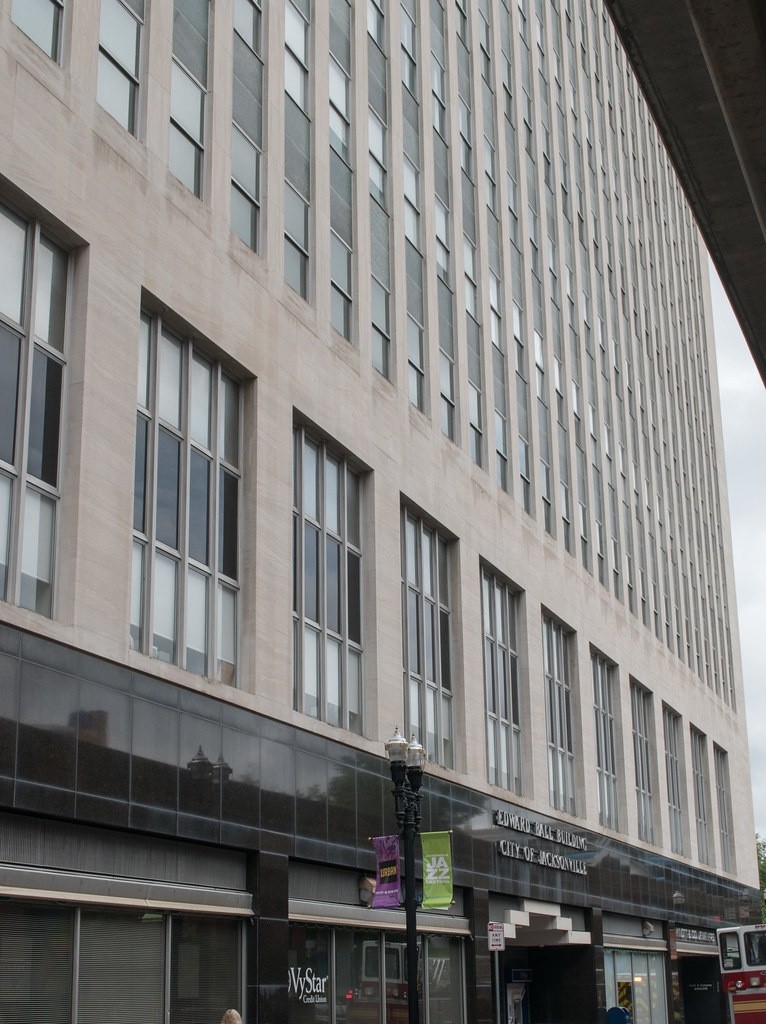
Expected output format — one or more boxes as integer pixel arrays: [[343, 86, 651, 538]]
[[385, 726, 429, 1024]]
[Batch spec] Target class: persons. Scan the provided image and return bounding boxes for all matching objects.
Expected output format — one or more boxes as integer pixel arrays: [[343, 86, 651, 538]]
[[221, 1009, 242, 1024]]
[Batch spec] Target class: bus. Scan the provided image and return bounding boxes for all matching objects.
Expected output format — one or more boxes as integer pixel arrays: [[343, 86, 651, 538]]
[[715, 923, 766, 1023]]
